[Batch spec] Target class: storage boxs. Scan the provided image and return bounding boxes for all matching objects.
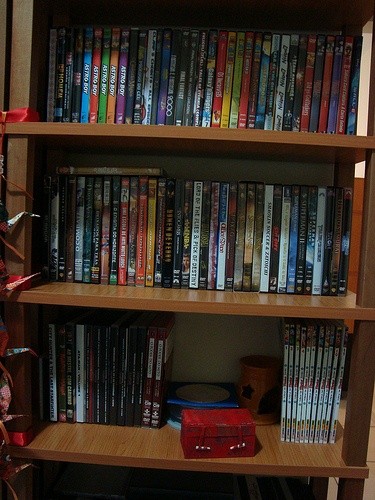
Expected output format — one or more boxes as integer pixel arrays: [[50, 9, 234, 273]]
[[180, 407, 256, 459]]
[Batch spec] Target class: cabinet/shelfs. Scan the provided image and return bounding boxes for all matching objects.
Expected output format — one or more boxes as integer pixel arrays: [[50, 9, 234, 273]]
[[5, 0, 375, 500]]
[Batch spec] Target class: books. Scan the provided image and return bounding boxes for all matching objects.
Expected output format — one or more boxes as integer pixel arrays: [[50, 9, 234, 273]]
[[39, 22, 363, 445]]
